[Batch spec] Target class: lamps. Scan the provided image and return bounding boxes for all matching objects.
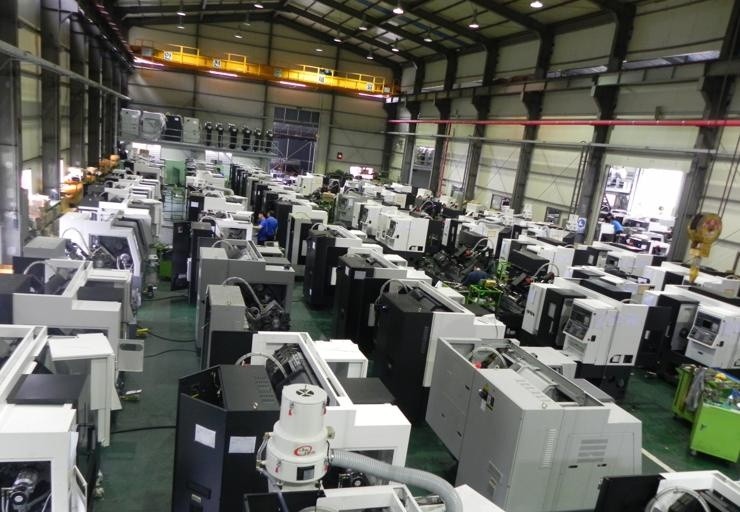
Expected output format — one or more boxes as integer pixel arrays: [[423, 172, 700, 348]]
[[175, 0, 187, 30], [469, 0, 479, 29], [233, 0, 264, 39], [315, 0, 432, 60]]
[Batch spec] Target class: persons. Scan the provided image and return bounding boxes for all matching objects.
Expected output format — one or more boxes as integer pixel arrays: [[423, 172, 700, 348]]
[[612, 165, 628, 190], [253, 210, 269, 245], [607, 164, 617, 186], [602, 213, 613, 224], [266, 210, 279, 241], [607, 216, 624, 244], [454, 260, 488, 289]]
